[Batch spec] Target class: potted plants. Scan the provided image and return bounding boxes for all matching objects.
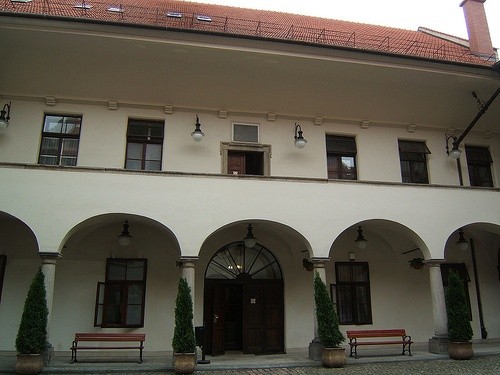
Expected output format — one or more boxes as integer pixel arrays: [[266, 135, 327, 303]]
[[13, 262, 50, 375], [444, 262, 476, 359], [171, 276, 197, 375], [312, 270, 347, 369]]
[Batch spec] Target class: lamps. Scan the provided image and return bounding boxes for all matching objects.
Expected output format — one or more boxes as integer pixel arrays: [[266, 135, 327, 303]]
[[293, 121, 308, 149], [353, 226, 368, 249], [445, 133, 463, 160], [117, 219, 133, 247], [0, 101, 11, 128], [457, 229, 470, 251], [243, 222, 257, 248], [191, 114, 205, 142]]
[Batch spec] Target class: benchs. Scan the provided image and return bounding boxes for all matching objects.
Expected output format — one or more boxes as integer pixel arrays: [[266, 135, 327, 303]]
[[70, 332, 147, 363], [345, 329, 414, 359]]
